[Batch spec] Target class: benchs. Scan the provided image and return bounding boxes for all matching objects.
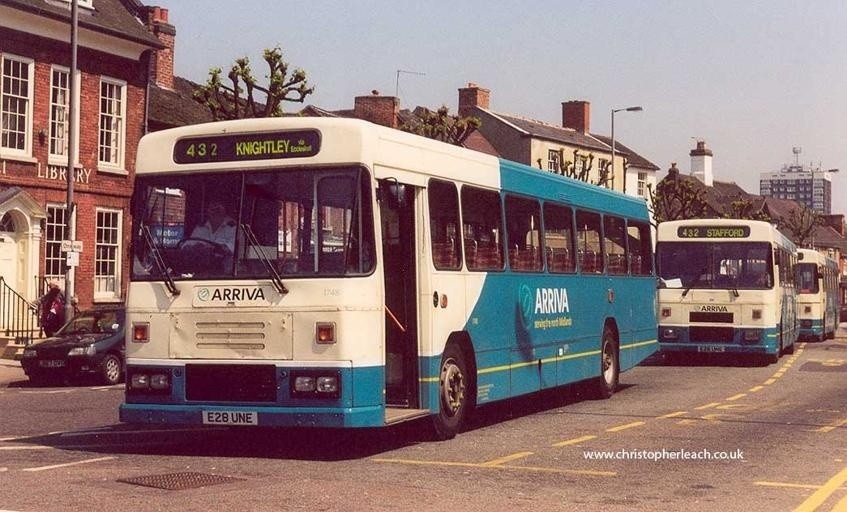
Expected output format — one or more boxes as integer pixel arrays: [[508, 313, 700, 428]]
[[301, 237, 642, 277]]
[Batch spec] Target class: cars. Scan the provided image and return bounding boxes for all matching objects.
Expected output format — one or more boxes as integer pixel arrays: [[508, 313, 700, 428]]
[[18, 302, 125, 387]]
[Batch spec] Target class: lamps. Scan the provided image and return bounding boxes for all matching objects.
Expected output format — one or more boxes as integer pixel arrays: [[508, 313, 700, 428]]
[[38, 128, 49, 138]]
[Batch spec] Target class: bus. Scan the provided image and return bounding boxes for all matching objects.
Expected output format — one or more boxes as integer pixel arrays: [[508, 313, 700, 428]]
[[650, 217, 801, 363], [793, 246, 842, 343], [107, 112, 664, 453]]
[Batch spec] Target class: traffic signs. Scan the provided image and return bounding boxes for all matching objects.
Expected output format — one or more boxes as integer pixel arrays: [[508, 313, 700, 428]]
[[61, 240, 84, 253]]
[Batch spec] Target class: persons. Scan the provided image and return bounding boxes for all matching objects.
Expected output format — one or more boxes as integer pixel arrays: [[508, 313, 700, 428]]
[[182, 197, 237, 257], [38, 287, 65, 337], [29, 281, 65, 315]]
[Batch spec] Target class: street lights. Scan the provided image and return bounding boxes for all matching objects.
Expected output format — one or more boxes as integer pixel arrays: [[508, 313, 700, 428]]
[[610, 104, 644, 190], [811, 168, 840, 250]]
